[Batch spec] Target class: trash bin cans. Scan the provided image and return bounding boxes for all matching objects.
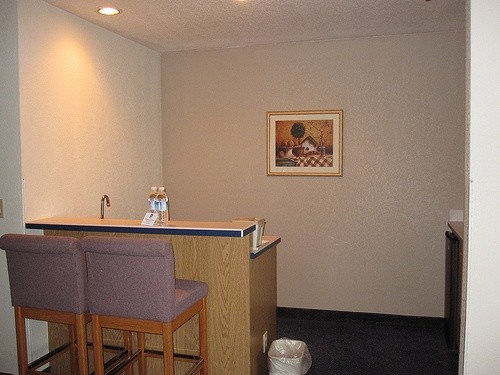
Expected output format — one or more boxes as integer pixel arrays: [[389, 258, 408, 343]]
[[268, 338, 306, 375]]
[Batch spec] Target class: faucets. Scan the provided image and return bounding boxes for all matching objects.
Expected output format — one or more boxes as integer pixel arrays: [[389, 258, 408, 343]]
[[100, 194, 110, 219]]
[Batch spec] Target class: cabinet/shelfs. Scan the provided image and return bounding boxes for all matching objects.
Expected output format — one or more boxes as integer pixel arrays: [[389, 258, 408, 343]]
[[443, 221, 463, 350]]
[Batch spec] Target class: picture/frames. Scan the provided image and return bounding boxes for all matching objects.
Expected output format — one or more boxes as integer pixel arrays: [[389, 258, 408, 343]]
[[267, 110, 344, 176]]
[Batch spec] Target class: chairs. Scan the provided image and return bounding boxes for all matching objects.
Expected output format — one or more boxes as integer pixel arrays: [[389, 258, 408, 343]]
[[0, 233, 133, 375], [81, 236, 208, 375]]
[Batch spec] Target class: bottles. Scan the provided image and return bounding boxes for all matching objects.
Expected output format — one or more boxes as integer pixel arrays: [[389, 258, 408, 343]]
[[155, 187, 169, 221], [148, 187, 158, 213]]
